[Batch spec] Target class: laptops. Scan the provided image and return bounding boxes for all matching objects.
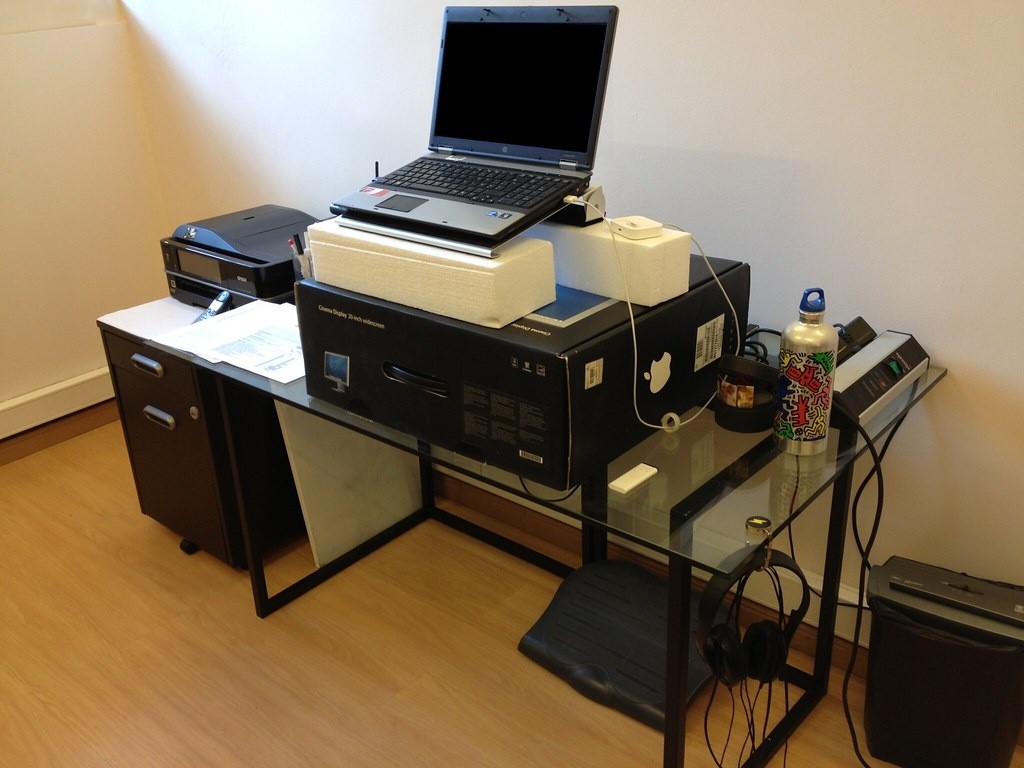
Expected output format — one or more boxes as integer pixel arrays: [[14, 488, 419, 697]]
[[330, 6, 619, 251]]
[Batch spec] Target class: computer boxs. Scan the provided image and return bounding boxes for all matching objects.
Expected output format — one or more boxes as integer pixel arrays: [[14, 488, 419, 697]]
[[863, 555, 1024, 768]]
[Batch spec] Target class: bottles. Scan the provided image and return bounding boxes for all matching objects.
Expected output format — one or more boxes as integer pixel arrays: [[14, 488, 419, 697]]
[[775, 287, 839, 455]]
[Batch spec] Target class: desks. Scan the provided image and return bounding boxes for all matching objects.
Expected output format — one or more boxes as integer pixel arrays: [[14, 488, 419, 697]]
[[143, 289, 947, 768]]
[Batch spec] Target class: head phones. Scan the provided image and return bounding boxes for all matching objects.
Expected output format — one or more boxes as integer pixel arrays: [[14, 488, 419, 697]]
[[694, 546, 810, 687]]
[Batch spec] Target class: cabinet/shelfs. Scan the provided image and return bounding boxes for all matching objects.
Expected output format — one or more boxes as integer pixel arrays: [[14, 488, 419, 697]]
[[97, 296, 306, 571]]
